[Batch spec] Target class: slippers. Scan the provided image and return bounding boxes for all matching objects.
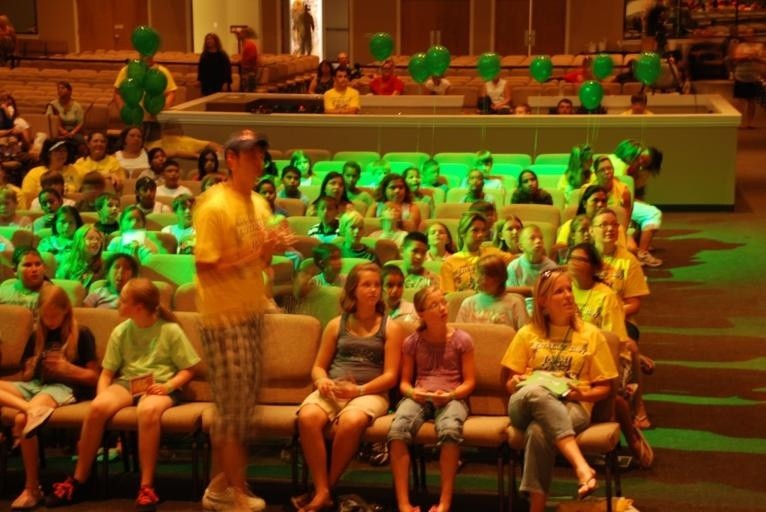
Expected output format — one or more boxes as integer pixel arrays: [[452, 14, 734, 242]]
[[292, 493, 314, 509], [578, 474, 599, 499], [632, 425, 654, 466], [634, 414, 651, 428], [300, 504, 334, 511], [640, 356, 656, 375]]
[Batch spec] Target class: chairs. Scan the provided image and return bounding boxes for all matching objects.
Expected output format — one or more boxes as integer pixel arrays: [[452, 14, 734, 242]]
[[0, 307, 33, 374], [104, 311, 205, 498], [504, 330, 622, 512], [299, 321, 419, 487], [443, 289, 479, 322], [496, 204, 560, 229], [146, 213, 182, 225], [1, 278, 85, 309], [366, 201, 429, 218], [286, 216, 321, 236], [413, 322, 518, 512], [350, 200, 366, 217], [433, 203, 471, 219], [302, 286, 343, 328], [401, 287, 421, 304], [300, 257, 371, 276], [173, 283, 200, 311], [270, 256, 294, 282], [520, 220, 556, 261], [418, 219, 461, 247], [383, 260, 444, 276], [87, 279, 174, 312], [142, 253, 198, 285], [2, 210, 123, 275], [363, 218, 384, 237], [1, 307, 127, 428], [201, 314, 321, 492], [273, 198, 305, 217], [561, 204, 627, 230]]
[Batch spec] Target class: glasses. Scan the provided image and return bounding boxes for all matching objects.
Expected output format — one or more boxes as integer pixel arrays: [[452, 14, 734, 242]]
[[85, 237, 103, 241], [593, 222, 620, 228], [423, 299, 448, 312]]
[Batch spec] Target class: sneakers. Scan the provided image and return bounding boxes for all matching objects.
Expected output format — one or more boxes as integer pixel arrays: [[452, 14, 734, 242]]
[[48, 477, 86, 502], [203, 483, 266, 511], [136, 485, 158, 510], [370, 442, 389, 466], [638, 253, 664, 267], [22, 406, 54, 438], [11, 489, 44, 507]]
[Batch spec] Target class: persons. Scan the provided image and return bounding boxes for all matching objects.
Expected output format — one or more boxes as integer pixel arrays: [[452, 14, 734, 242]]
[[2, 1, 666, 511], [720, 23, 764, 130]]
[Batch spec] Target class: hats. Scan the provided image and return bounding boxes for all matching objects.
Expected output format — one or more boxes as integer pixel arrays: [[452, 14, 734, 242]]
[[224, 132, 270, 153], [136, 176, 155, 202]]
